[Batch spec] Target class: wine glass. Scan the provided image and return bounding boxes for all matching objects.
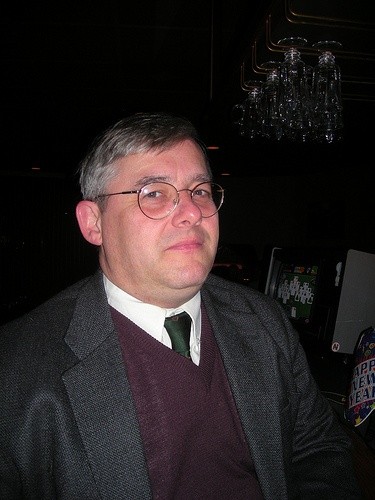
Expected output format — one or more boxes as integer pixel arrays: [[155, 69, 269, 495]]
[[232, 37, 344, 144]]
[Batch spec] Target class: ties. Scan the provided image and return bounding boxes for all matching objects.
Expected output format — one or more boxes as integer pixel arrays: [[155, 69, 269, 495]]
[[164, 311, 193, 363]]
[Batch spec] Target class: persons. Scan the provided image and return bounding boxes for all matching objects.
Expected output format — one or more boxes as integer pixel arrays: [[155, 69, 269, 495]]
[[0, 111, 358, 500]]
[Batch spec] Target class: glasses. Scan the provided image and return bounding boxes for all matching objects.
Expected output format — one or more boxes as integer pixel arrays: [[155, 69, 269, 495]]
[[95, 180, 225, 221]]
